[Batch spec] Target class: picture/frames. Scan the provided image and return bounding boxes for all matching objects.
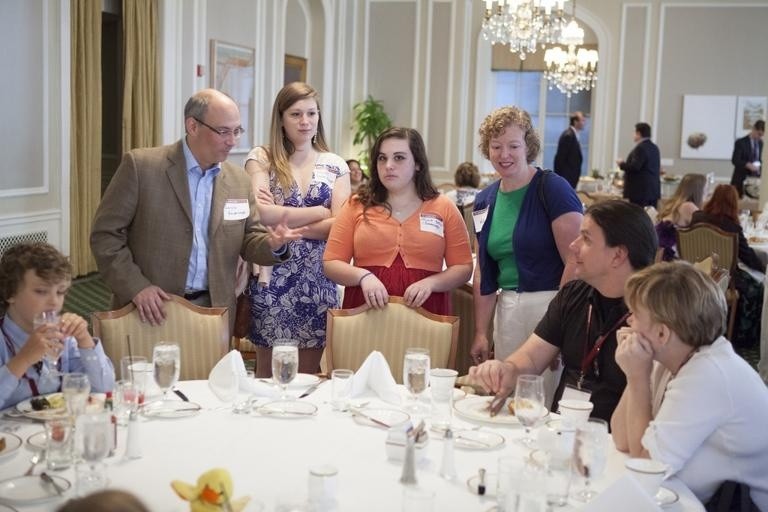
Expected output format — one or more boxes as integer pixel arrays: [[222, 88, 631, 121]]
[[210, 39, 256, 154], [285, 53, 307, 86]]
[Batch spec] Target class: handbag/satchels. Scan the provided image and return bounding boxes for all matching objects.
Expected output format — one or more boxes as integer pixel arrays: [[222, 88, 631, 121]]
[[233, 288, 251, 338]]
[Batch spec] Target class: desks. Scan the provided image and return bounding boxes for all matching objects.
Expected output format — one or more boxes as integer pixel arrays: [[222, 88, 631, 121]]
[[660, 174, 732, 204]]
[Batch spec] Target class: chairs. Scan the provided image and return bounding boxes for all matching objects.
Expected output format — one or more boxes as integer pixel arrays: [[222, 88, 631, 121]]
[[452, 282, 497, 377], [673, 222, 739, 341], [90, 294, 231, 381], [576, 190, 597, 208], [693, 250, 720, 275], [711, 265, 732, 294], [436, 182, 456, 191], [325, 296, 461, 384], [455, 201, 476, 253]]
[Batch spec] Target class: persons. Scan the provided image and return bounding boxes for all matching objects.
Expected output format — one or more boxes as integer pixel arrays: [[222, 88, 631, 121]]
[[656, 173, 767, 344], [730, 119, 766, 215], [245, 80, 350, 379], [611, 257, 768, 512], [468, 106, 586, 411], [1, 241, 116, 411], [616, 123, 661, 209], [89, 89, 310, 350], [322, 125, 482, 316], [469, 197, 657, 432]]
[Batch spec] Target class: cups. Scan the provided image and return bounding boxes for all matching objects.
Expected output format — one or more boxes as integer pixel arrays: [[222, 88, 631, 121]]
[[431, 369, 458, 400], [307, 468, 338, 508], [625, 458, 673, 497], [42, 416, 76, 469], [330, 370, 353, 414], [556, 397, 593, 431]]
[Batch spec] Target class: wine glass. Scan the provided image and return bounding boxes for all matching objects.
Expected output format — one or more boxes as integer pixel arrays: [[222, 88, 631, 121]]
[[152, 342, 180, 401], [273, 339, 299, 399], [738, 209, 764, 245], [514, 376, 548, 447], [401, 347, 433, 413], [32, 310, 67, 379], [66, 353, 153, 483]]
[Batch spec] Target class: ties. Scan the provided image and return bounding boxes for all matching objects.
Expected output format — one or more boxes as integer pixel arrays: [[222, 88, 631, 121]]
[[752, 138, 758, 177]]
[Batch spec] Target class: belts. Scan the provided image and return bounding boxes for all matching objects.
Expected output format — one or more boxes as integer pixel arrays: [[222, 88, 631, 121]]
[[185, 291, 208, 300]]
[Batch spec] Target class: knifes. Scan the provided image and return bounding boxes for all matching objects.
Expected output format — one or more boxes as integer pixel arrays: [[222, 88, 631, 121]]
[[39, 471, 64, 497], [24, 454, 40, 477]]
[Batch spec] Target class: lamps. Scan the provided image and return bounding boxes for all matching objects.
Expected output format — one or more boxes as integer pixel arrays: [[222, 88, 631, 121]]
[[543, 0, 600, 99], [481, 0, 569, 61]]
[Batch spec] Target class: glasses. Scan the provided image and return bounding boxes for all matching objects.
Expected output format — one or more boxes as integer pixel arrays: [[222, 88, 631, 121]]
[[193, 116, 245, 140]]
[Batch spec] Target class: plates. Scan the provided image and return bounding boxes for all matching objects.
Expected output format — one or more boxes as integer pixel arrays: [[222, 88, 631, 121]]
[[442, 430, 505, 452], [452, 395, 551, 425], [258, 401, 320, 420], [0, 429, 23, 457], [27, 429, 48, 450], [654, 486, 680, 506], [356, 410, 410, 427], [142, 401, 202, 419], [271, 374, 320, 388], [0, 475, 71, 503], [16, 391, 71, 420], [420, 389, 467, 400]]
